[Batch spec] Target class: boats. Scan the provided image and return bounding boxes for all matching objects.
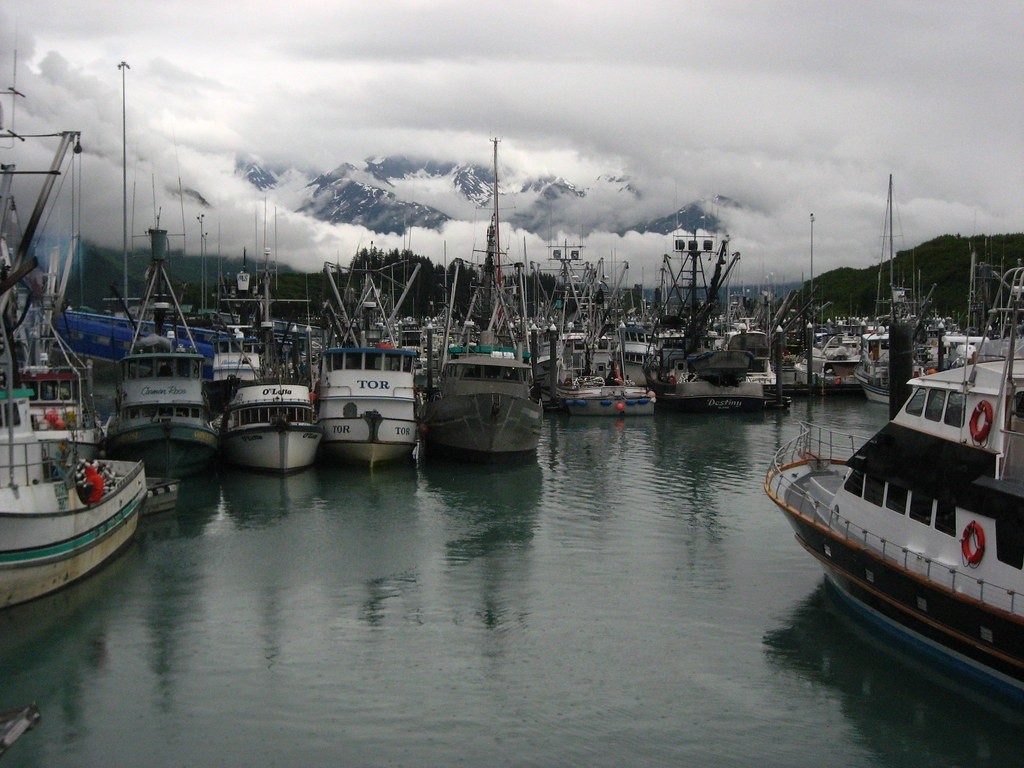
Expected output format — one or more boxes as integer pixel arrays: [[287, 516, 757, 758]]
[[0, 59, 1023, 693]]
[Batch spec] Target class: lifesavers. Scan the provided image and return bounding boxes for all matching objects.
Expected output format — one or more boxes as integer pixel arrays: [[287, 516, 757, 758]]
[[960, 521, 985, 564], [968, 399, 994, 442]]
